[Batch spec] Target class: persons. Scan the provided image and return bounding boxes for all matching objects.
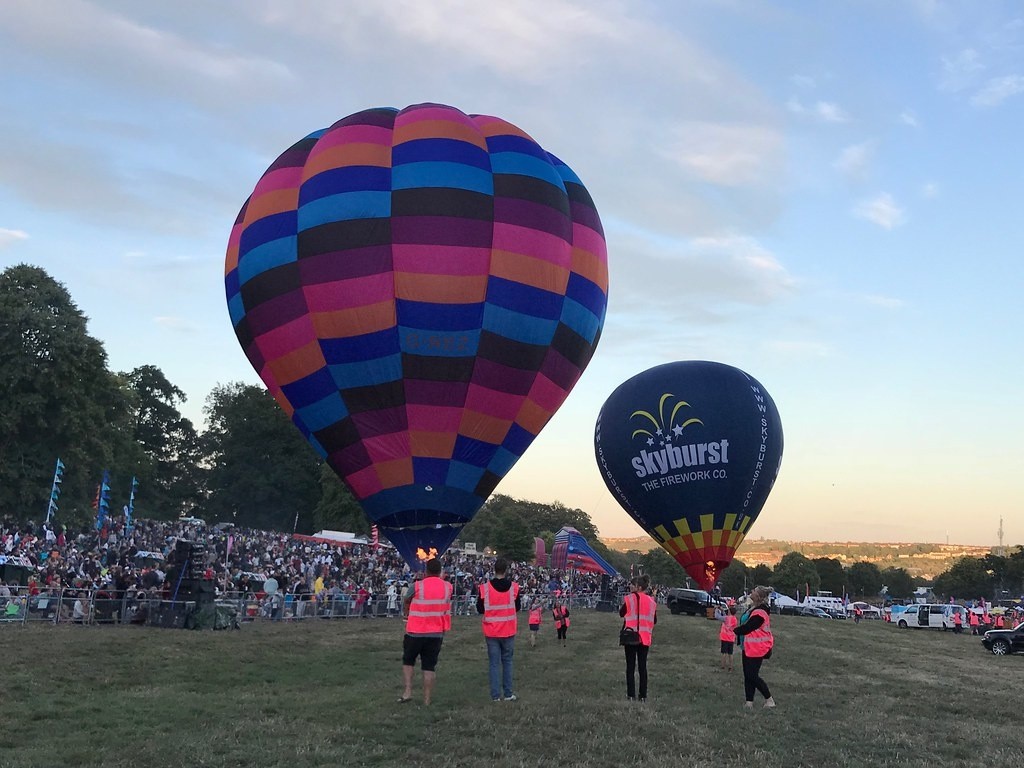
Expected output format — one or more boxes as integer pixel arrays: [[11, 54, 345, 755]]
[[714, 605, 737, 671], [0, 516, 669, 627], [617, 574, 657, 702], [854, 605, 861, 624], [553, 600, 571, 647], [731, 585, 776, 709], [398, 559, 453, 707], [476, 558, 520, 701], [528, 597, 546, 649], [954, 607, 1024, 635]]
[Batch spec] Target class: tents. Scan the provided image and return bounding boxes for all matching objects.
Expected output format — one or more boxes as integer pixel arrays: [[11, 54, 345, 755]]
[[770, 591, 802, 608]]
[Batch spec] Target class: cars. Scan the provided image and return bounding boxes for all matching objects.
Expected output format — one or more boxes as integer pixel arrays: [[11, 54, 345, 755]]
[[720, 595, 896, 621], [981, 622, 1024, 656], [717, 601, 728, 616], [799, 606, 832, 620]]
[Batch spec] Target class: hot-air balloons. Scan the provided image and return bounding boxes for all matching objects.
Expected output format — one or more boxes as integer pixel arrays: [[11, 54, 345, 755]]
[[224, 102, 610, 621], [593, 360, 784, 621]]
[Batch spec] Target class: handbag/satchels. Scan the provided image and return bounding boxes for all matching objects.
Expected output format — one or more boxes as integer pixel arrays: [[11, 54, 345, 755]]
[[619, 627, 643, 646]]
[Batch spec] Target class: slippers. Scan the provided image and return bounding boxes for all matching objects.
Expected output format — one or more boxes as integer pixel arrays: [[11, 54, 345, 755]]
[[398, 697, 412, 703]]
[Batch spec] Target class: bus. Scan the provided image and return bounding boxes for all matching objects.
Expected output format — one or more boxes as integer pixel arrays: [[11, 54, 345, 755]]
[[969, 598, 1024, 617]]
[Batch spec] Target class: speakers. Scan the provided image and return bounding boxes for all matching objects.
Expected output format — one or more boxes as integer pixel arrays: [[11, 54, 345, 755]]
[[596, 574, 616, 612], [160, 539, 216, 632]]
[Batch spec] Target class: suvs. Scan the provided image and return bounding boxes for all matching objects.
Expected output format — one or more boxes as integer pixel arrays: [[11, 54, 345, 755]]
[[667, 586, 721, 617]]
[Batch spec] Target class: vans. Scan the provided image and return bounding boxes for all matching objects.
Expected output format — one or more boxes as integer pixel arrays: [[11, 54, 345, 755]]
[[896, 603, 971, 632]]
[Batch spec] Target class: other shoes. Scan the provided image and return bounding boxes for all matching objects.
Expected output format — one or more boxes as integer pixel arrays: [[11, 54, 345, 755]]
[[494, 699, 500, 701], [745, 701, 754, 707], [505, 695, 516, 701], [765, 697, 776, 707]]
